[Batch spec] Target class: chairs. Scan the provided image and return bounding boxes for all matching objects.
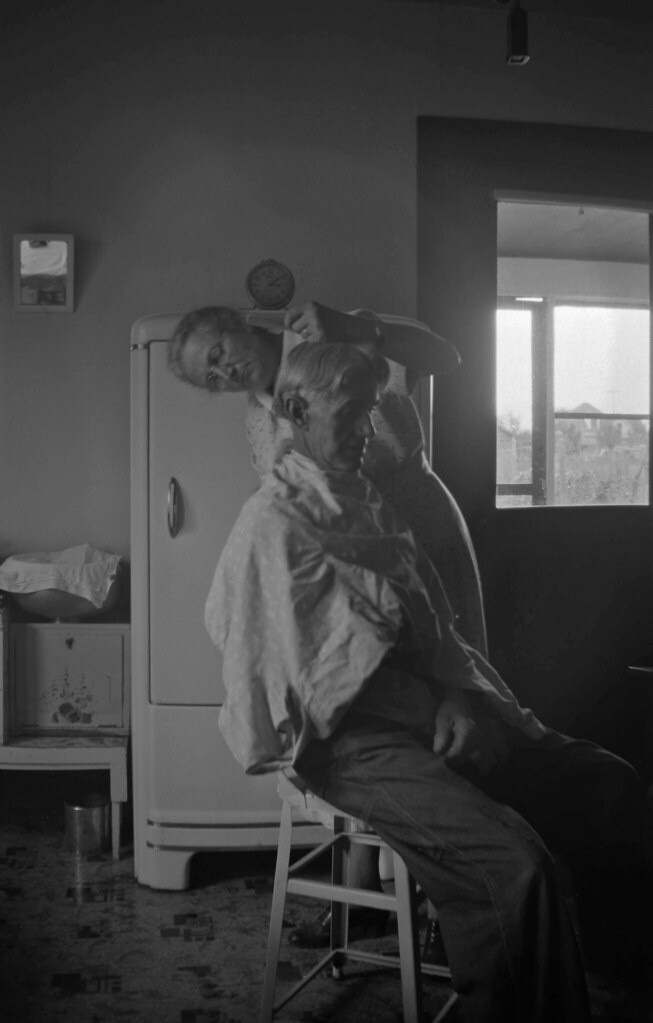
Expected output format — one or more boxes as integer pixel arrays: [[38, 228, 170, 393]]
[[0, 623, 134, 862]]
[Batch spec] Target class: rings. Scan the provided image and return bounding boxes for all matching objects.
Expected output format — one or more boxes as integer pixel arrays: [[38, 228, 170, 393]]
[[303, 329, 311, 336]]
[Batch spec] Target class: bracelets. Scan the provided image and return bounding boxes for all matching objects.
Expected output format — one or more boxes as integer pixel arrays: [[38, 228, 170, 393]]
[[351, 309, 386, 349]]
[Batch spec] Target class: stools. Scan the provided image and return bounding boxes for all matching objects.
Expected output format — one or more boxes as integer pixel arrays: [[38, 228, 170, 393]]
[[264, 777, 461, 1022]]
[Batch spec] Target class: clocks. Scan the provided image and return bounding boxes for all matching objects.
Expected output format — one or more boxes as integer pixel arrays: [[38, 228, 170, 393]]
[[244, 260, 295, 310]]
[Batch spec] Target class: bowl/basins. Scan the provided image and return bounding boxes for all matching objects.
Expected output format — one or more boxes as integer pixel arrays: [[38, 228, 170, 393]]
[[10, 553, 124, 624]]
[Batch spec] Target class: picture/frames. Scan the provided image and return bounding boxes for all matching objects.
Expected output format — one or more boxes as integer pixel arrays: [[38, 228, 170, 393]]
[[12, 234, 74, 315]]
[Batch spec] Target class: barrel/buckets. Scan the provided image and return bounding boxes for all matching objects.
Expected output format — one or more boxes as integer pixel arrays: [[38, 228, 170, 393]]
[[64, 797, 111, 856]]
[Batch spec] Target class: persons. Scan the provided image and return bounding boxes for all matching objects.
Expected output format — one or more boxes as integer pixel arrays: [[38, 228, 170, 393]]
[[205, 345, 653, 1023], [167, 300, 487, 663]]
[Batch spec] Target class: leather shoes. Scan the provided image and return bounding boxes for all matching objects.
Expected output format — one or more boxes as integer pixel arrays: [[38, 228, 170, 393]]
[[288, 904, 391, 948], [419, 919, 450, 981]]
